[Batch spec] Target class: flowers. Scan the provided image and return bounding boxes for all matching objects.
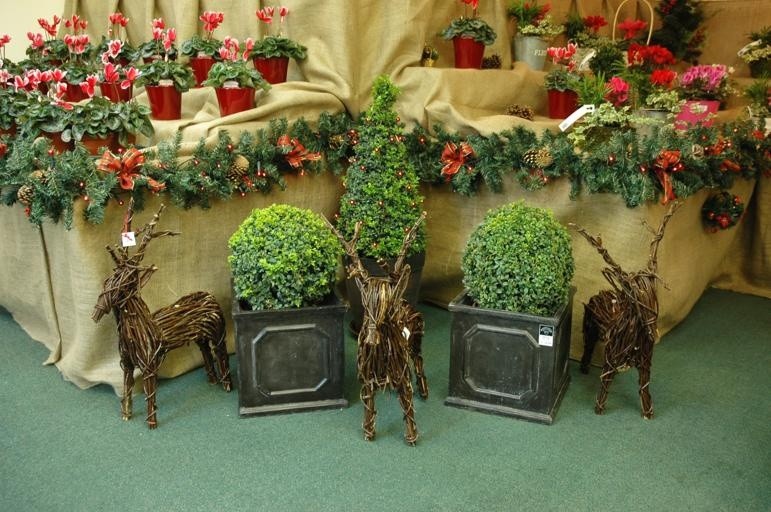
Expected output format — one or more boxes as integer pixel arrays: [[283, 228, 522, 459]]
[[582, 11, 733, 110], [540, 41, 588, 92], [102, 62, 135, 88], [131, 18, 178, 60], [201, 36, 271, 88], [250, 4, 308, 60], [0, 9, 131, 63], [0, 66, 50, 93], [503, 0, 564, 39], [181, 10, 229, 61], [50, 62, 96, 102], [437, 0, 498, 46]]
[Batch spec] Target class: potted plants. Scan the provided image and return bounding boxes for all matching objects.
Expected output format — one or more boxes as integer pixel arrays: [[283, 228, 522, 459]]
[[224, 204, 353, 416], [135, 60, 192, 120], [0, 95, 154, 154], [442, 200, 573, 427], [330, 78, 426, 340]]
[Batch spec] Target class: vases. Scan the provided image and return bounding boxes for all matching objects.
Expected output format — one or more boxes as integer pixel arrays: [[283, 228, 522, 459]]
[[451, 37, 485, 68], [511, 36, 548, 70], [252, 56, 288, 84], [188, 55, 218, 87], [214, 88, 256, 117], [544, 85, 579, 119], [99, 83, 132, 103], [140, 54, 176, 64], [60, 78, 89, 102], [673, 100, 720, 131]]
